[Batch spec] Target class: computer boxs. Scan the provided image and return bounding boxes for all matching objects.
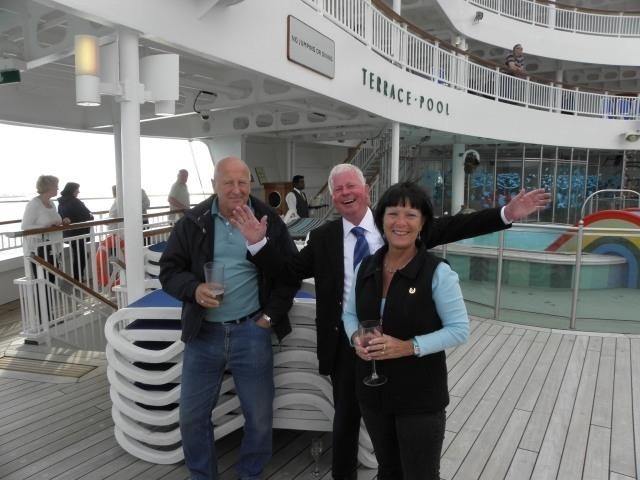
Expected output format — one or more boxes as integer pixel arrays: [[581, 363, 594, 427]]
[[199, 110, 211, 120]]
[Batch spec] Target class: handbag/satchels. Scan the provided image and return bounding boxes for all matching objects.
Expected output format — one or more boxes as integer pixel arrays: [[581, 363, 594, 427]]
[[411, 339, 420, 357], [262, 313, 274, 326]]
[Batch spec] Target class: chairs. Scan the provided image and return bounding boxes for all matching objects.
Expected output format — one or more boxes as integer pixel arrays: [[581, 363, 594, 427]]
[[103, 227, 380, 472]]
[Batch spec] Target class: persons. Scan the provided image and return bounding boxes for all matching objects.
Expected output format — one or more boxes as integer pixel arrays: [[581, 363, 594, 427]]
[[166, 169, 191, 225], [141, 189, 153, 246], [156, 155, 302, 479], [342, 180, 471, 480], [56, 181, 94, 284], [504, 42, 529, 75], [19, 174, 64, 328], [105, 185, 119, 235], [285, 175, 311, 219], [227, 161, 554, 480]]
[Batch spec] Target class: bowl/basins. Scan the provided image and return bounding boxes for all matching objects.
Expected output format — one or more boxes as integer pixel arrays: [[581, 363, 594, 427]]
[[383, 254, 414, 274]]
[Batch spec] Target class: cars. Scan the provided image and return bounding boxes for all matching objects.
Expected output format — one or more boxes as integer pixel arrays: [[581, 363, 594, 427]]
[[203, 262, 227, 307]]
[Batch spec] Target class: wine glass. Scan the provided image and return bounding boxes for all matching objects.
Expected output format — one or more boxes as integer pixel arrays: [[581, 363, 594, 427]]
[[380, 352, 387, 355], [200, 300, 205, 305], [381, 343, 387, 350]]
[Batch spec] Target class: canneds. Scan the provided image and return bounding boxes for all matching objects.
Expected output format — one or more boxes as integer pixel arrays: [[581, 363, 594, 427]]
[[97, 237, 125, 287]]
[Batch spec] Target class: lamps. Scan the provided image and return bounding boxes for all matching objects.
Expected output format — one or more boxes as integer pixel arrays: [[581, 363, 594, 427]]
[[138, 53, 181, 116], [71, 33, 124, 108]]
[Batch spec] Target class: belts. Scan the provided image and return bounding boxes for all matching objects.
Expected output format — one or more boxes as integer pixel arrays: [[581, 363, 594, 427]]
[[355, 320, 390, 388], [307, 437, 326, 478]]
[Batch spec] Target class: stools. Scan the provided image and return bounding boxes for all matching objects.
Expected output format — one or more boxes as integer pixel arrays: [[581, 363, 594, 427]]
[[201, 308, 262, 325]]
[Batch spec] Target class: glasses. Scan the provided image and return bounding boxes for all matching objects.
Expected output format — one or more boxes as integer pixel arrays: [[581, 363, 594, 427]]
[[71, 33, 124, 108], [138, 53, 181, 116]]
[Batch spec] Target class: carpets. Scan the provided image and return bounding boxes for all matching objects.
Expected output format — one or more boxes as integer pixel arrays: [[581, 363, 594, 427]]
[[301, 192, 306, 201], [351, 226, 371, 273]]
